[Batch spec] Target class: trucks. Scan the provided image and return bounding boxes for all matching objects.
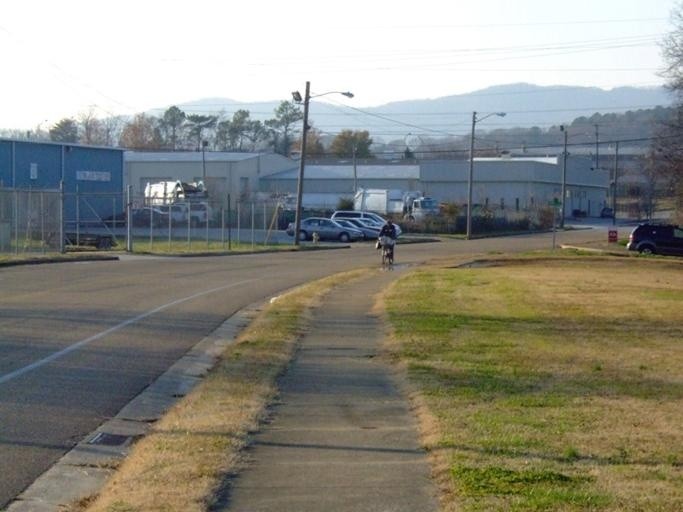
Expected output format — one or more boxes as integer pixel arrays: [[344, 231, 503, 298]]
[[355, 188, 440, 223]]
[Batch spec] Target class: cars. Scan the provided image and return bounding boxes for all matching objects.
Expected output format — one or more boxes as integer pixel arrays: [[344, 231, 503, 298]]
[[626, 221, 683, 257], [107, 201, 211, 229], [287, 210, 402, 242]]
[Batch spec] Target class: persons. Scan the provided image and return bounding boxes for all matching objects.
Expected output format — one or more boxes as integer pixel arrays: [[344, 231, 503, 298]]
[[378, 219, 396, 265]]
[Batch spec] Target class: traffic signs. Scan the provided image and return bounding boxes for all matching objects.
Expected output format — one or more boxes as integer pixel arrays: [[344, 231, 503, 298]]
[[546, 200, 561, 205]]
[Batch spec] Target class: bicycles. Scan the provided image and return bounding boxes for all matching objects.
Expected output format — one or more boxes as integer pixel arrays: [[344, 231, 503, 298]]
[[377, 240, 394, 265]]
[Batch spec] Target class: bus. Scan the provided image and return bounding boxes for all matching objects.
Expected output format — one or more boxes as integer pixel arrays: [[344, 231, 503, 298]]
[[144, 181, 208, 206]]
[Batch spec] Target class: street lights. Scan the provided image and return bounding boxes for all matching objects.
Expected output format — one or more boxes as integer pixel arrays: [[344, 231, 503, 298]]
[[467, 111, 506, 239], [559, 125, 584, 228], [293, 81, 353, 246], [201, 141, 209, 183]]
